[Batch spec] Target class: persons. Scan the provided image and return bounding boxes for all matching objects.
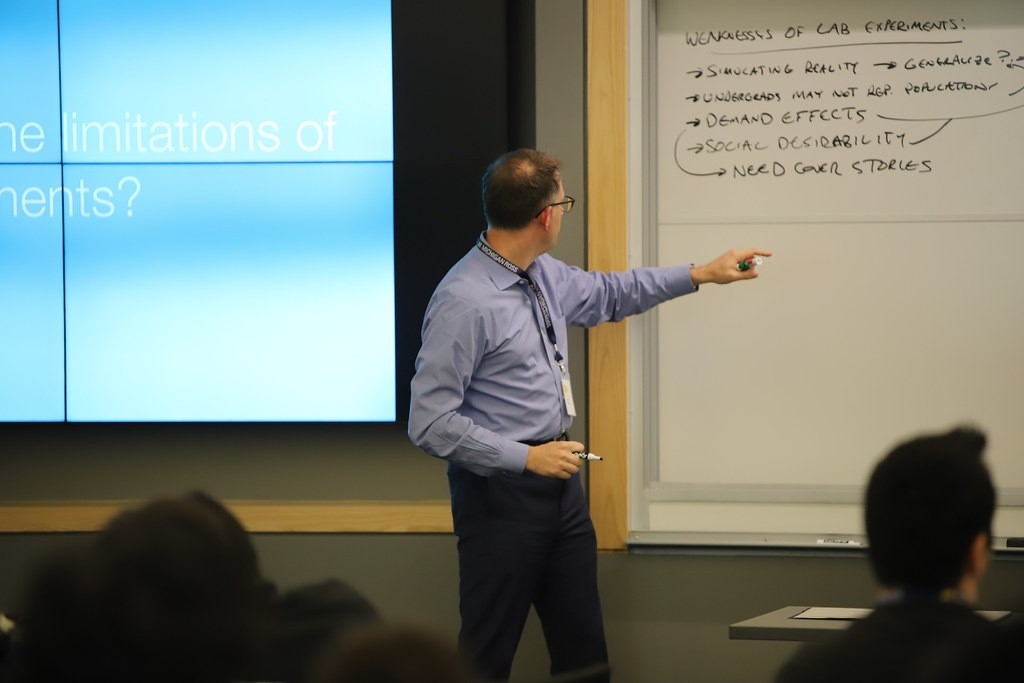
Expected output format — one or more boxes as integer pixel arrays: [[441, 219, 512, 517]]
[[407, 148, 772, 683], [776, 427, 1024, 683]]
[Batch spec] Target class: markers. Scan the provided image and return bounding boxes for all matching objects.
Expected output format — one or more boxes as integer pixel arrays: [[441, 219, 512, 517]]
[[735, 260, 749, 271], [745, 256, 763, 266], [815, 537, 864, 548], [573, 451, 604, 462]]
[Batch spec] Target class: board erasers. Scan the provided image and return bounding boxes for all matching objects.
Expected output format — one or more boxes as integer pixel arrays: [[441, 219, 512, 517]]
[[1006, 537, 1024, 548]]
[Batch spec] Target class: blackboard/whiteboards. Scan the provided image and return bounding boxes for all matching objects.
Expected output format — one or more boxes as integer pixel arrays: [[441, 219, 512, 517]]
[[622, 0, 1024, 555]]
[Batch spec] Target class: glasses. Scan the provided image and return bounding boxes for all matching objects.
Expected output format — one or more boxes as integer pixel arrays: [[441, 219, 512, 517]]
[[533, 195, 574, 221]]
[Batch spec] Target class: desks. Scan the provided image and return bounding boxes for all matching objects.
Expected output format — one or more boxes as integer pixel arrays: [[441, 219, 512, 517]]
[[727, 604, 1011, 642]]
